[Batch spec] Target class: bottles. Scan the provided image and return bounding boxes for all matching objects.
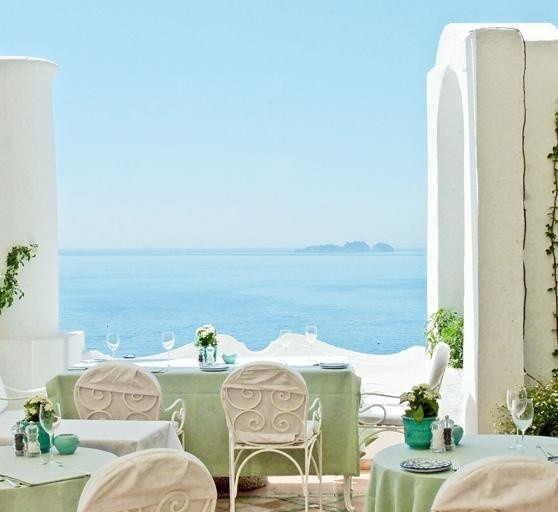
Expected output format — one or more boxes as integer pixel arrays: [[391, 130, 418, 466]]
[[430, 415, 454, 453], [13, 419, 41, 457], [197, 344, 214, 366]]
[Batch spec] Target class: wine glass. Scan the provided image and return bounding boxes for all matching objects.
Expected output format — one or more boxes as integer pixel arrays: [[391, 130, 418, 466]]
[[506, 386, 534, 453], [305, 326, 317, 361], [106, 332, 120, 360], [38, 402, 63, 467], [280, 330, 295, 366], [161, 331, 175, 372]]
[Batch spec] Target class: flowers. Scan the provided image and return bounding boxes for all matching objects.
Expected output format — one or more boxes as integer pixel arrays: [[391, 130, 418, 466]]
[[194, 324, 217, 348]]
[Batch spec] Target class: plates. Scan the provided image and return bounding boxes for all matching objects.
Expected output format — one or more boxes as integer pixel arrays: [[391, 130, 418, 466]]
[[400, 458, 451, 472], [320, 363, 349, 369]]
